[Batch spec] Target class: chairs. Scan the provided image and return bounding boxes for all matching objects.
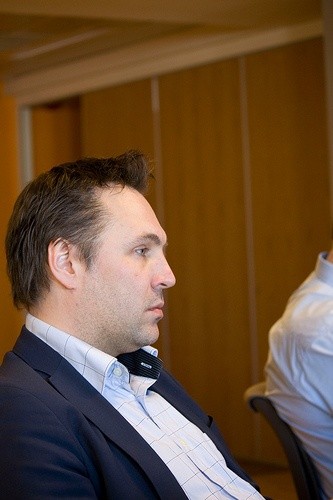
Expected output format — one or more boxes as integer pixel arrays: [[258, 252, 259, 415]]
[[243, 384, 322, 500]]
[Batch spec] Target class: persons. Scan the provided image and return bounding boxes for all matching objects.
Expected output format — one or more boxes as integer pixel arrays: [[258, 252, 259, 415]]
[[0, 149, 272, 500], [265, 246, 333, 500]]
